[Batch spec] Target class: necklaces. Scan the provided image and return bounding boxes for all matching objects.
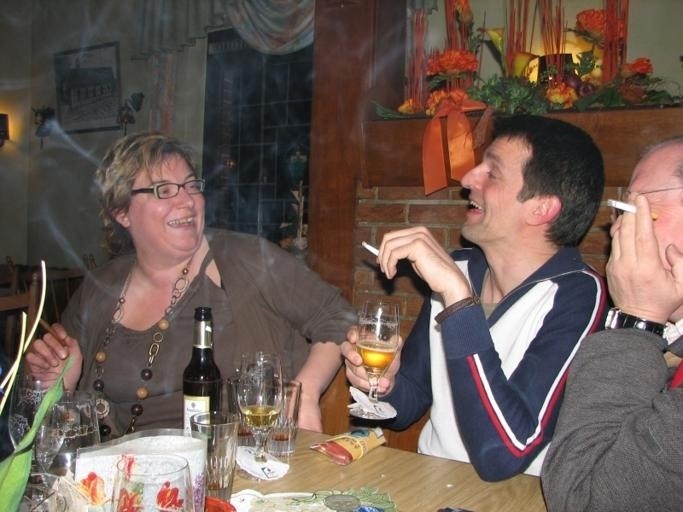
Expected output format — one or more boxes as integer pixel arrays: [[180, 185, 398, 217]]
[[94, 249, 193, 444]]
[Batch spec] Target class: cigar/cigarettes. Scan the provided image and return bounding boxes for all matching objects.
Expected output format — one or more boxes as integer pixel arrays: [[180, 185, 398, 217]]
[[362, 241, 380, 256], [39, 319, 67, 349], [607, 199, 660, 223]]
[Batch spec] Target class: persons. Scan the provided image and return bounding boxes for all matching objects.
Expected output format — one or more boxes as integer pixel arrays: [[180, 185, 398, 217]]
[[21, 129, 355, 451], [540, 135, 682, 512], [340, 114, 612, 483]]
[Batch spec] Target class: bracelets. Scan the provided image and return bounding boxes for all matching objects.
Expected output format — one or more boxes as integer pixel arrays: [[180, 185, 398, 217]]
[[434, 294, 480, 325], [604, 307, 663, 337]]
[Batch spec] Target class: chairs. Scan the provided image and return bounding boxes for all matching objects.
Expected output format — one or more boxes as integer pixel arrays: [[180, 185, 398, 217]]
[[1, 252, 99, 359]]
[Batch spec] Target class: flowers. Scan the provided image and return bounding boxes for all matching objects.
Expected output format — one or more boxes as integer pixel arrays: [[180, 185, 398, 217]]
[[367, 0, 683, 120]]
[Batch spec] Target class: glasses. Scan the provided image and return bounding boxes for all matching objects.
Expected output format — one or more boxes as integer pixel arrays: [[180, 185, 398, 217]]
[[130, 179, 207, 200], [619, 186, 681, 214]]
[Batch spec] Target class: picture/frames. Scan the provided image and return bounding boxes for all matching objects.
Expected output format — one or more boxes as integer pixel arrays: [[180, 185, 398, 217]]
[[53, 39, 125, 136]]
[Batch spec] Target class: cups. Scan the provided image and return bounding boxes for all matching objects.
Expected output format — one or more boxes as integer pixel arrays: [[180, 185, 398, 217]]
[[187, 409, 241, 505], [109, 451, 193, 512]]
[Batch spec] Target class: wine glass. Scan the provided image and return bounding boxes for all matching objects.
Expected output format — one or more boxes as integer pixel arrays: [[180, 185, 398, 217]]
[[348, 294, 400, 425], [8, 370, 101, 512], [225, 351, 302, 464]]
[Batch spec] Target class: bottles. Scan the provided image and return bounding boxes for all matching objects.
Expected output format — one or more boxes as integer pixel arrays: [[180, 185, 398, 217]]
[[181, 306, 223, 453]]
